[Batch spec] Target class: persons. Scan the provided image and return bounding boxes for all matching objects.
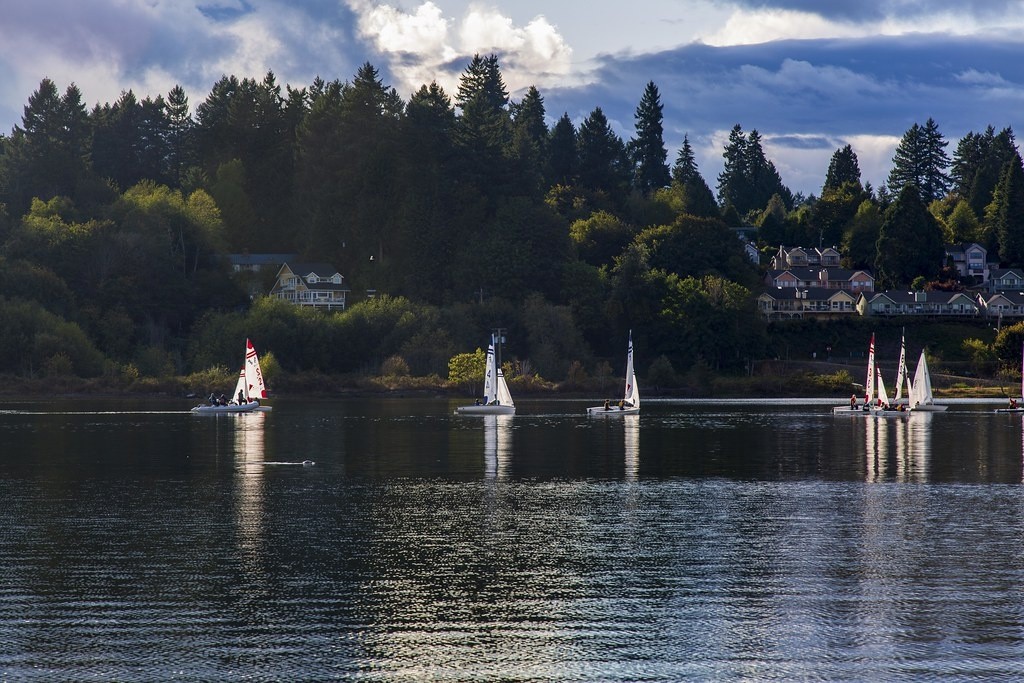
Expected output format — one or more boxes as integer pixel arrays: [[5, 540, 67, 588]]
[[238, 389, 247, 405], [619, 400, 625, 410], [881, 402, 904, 411], [219, 393, 228, 406], [604, 399, 613, 411], [209, 393, 219, 407], [851, 394, 858, 410], [1008, 396, 1018, 409]]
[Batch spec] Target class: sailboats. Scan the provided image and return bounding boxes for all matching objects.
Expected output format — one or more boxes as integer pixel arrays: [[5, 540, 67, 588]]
[[586, 329, 641, 416], [454, 328, 516, 415], [832, 327, 950, 415], [231, 338, 274, 411]]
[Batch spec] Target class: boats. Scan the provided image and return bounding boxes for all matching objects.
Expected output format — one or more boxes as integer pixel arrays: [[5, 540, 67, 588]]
[[993, 406, 1024, 412], [191, 400, 260, 413]]
